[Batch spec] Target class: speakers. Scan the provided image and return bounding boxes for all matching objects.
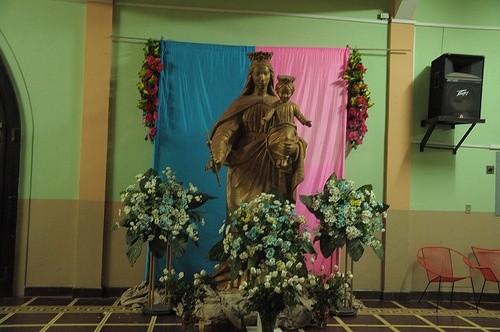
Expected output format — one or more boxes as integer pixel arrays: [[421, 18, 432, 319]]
[[428, 53, 485, 119]]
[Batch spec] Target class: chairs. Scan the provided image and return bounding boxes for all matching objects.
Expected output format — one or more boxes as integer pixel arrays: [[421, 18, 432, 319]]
[[464, 246, 500, 309], [416, 247, 479, 314]]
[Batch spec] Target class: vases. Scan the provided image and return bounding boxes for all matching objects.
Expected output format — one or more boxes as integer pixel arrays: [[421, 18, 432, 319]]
[[318, 314, 327, 332], [180, 311, 195, 332]]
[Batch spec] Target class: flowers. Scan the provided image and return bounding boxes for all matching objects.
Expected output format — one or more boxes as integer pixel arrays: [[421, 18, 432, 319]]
[[111, 166, 220, 268], [205, 193, 318, 284], [300, 172, 391, 262], [135, 36, 164, 145], [341, 48, 377, 153], [237, 260, 305, 332], [157, 263, 221, 314], [305, 257, 354, 332]]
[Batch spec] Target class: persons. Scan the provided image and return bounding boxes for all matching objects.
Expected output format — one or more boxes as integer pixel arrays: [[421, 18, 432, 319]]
[[261, 74, 312, 166], [204, 51, 307, 289]]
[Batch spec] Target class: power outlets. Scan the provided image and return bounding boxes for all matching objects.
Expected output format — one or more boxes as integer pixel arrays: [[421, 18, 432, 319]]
[[466, 205, 472, 214]]
